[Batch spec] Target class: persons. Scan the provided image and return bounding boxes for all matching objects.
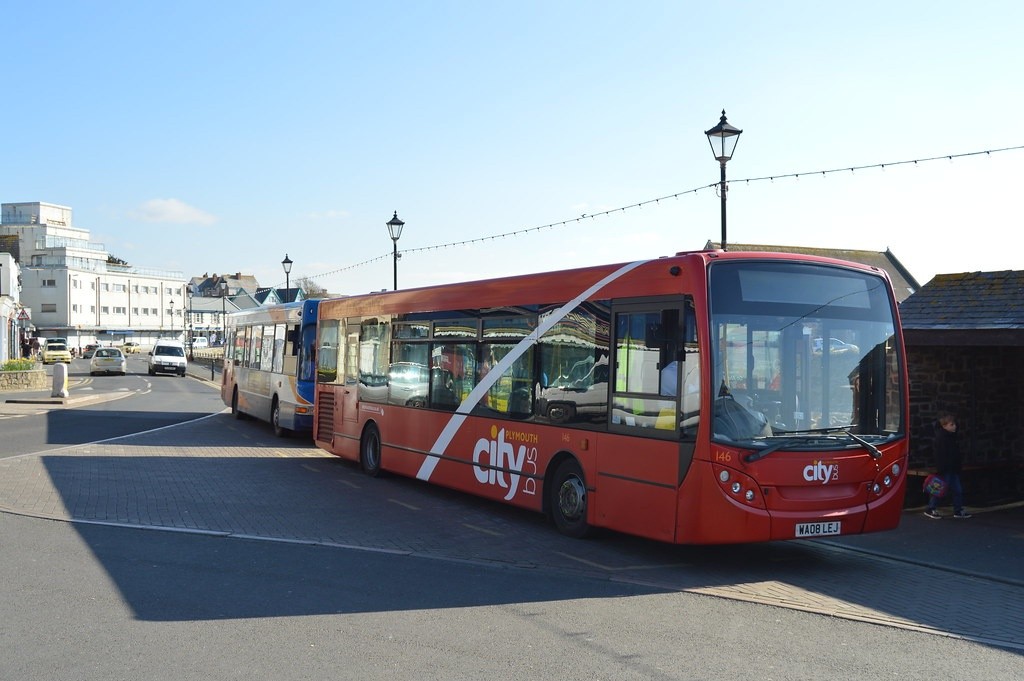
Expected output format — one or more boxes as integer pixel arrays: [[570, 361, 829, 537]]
[[660, 344, 699, 408], [923, 413, 972, 519], [210, 331, 216, 347], [483, 352, 501, 407], [21, 340, 33, 360], [32, 338, 42, 360]]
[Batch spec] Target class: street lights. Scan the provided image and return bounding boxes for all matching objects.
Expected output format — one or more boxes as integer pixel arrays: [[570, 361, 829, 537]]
[[704, 108, 743, 252], [386, 209, 406, 290], [281, 253, 294, 303]]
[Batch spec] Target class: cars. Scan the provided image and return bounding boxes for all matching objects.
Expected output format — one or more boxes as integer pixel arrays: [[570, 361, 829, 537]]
[[358, 358, 451, 408], [538, 358, 608, 427], [41, 343, 73, 364], [82, 344, 99, 359], [89, 347, 127, 376], [123, 341, 142, 354]]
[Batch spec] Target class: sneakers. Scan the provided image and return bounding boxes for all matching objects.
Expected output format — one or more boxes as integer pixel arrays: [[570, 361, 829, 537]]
[[924, 508, 944, 520], [953, 511, 972, 519]]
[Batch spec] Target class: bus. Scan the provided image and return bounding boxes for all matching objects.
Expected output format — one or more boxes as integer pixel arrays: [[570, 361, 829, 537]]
[[220, 298, 329, 438], [312, 248, 910, 551]]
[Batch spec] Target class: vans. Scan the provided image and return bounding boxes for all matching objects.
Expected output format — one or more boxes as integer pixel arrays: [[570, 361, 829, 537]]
[[147, 339, 187, 378], [41, 337, 68, 349], [185, 337, 208, 349]]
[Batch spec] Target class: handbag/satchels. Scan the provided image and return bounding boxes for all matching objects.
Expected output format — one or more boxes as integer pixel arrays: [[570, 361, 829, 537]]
[[923, 472, 948, 499]]
